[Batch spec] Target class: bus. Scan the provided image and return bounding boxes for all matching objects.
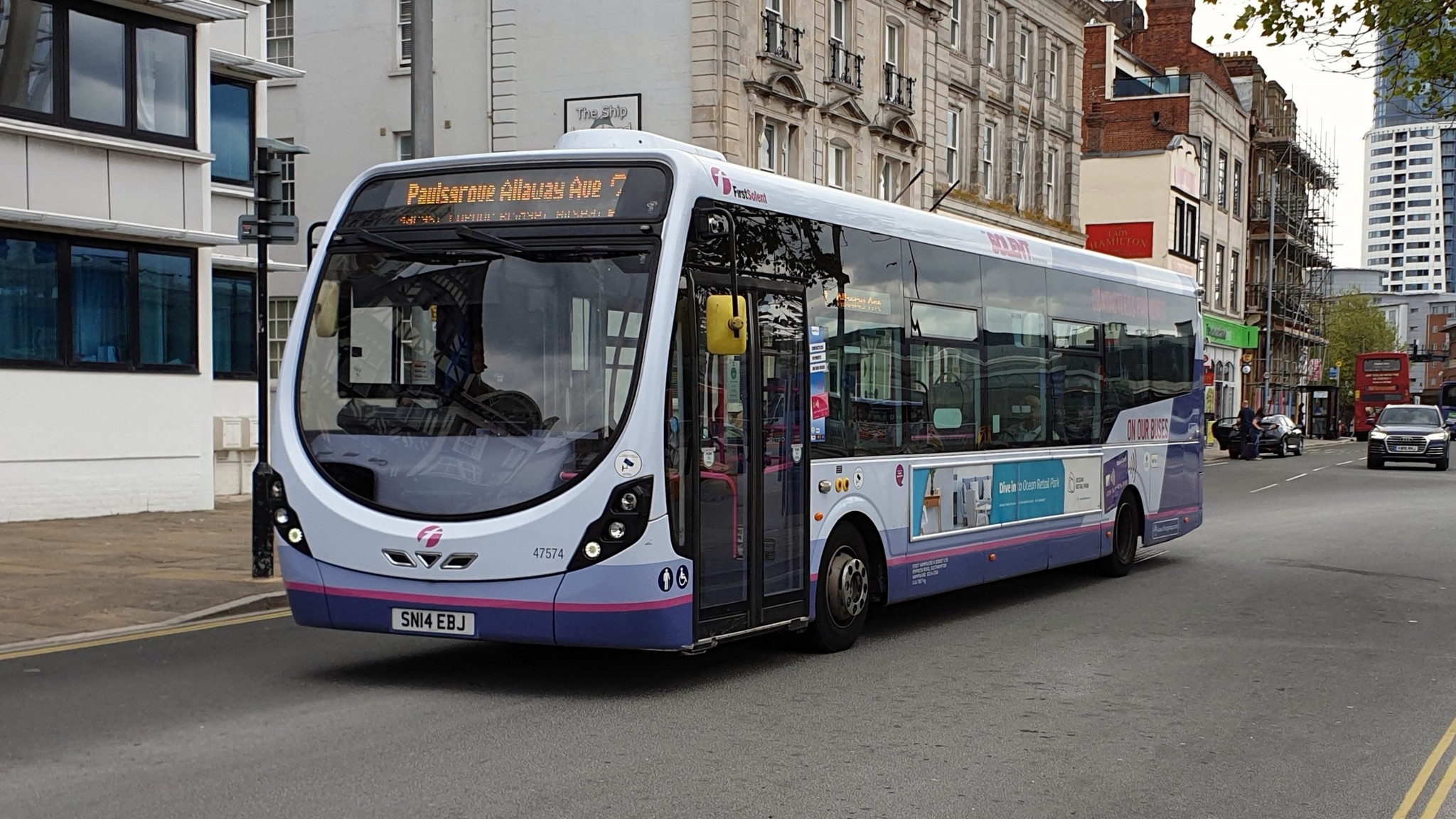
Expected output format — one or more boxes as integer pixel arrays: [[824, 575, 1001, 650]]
[[1354, 352, 1411, 442], [1440, 381, 1456, 440], [266, 126, 1203, 657]]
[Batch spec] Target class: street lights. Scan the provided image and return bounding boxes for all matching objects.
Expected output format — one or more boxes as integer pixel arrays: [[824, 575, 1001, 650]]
[[1264, 163, 1293, 414]]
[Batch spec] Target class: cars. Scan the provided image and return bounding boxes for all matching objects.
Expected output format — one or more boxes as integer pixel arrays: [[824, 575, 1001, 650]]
[[1211, 415, 1305, 458]]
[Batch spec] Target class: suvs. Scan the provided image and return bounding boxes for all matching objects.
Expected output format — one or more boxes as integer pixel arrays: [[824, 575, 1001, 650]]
[[1365, 403, 1451, 471]]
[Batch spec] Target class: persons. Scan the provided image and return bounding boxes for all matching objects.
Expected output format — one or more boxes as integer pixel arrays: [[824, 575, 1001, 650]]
[[994, 394, 1059, 449], [440, 336, 513, 397], [1297, 402, 1307, 425], [1237, 400, 1254, 460], [1312, 400, 1328, 440], [1249, 405, 1266, 461]]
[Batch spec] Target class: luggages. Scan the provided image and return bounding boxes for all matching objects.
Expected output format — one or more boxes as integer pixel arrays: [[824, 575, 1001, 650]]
[[1239, 429, 1262, 461]]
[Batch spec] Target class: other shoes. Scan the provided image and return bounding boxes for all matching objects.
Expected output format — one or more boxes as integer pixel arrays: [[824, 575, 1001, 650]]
[[1250, 456, 1263, 461]]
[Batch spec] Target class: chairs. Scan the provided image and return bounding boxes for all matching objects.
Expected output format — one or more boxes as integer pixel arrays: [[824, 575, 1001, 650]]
[[909, 342, 1183, 453], [1420, 411, 1432, 422]]
